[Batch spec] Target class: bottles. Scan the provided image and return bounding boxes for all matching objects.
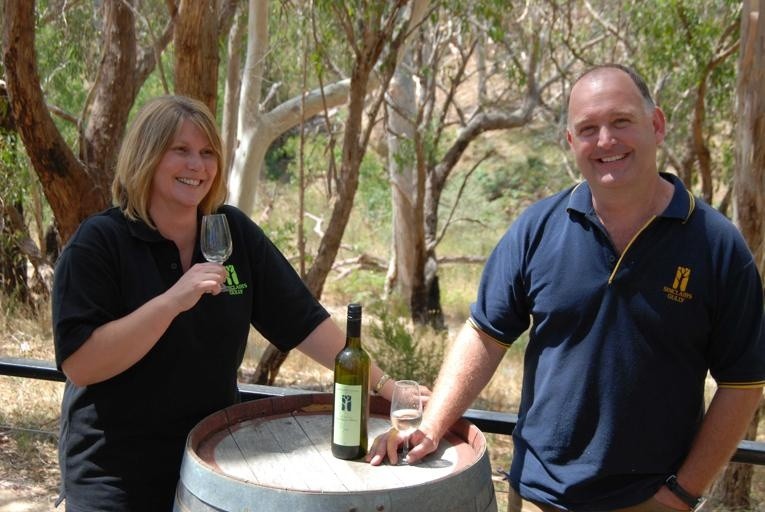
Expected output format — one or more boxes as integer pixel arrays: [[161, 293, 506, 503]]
[[331, 303, 371, 461]]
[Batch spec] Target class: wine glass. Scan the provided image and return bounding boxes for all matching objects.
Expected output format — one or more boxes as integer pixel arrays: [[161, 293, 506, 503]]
[[389, 380, 422, 465], [199, 213, 233, 293]]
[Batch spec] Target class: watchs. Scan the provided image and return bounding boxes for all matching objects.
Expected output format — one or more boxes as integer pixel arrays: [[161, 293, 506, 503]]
[[666, 474, 707, 512]]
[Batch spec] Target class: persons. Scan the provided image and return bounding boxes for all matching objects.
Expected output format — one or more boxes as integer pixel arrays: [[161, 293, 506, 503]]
[[365, 64, 765, 512], [51, 97, 434, 512]]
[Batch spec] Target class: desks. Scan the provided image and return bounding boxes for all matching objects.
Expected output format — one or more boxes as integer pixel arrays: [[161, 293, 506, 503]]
[[171, 393, 499, 512]]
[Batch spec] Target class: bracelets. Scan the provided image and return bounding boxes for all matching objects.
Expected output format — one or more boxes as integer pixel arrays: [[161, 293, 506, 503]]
[[374, 373, 391, 393]]
